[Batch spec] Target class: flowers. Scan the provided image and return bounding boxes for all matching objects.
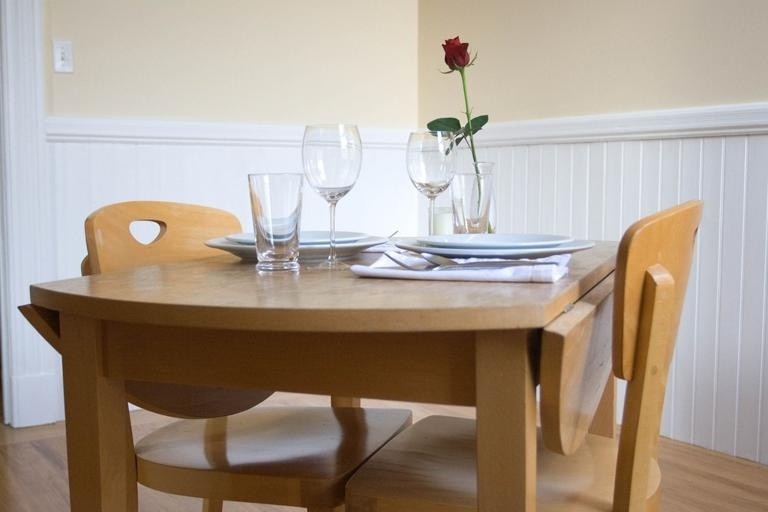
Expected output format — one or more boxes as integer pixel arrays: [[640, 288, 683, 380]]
[[426, 37, 489, 212]]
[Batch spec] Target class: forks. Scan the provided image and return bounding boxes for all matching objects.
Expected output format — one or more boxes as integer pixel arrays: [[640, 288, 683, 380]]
[[381, 251, 561, 271]]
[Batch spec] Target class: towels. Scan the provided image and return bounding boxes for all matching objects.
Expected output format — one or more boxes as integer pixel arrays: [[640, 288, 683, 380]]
[[351, 249, 572, 281]]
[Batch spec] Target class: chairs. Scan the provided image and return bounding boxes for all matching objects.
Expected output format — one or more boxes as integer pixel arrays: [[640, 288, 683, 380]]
[[344, 199, 703, 512], [82, 201, 412, 512]]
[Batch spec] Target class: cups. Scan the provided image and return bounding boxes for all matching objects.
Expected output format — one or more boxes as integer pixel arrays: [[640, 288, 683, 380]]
[[452, 161, 495, 234], [248, 172, 302, 272]]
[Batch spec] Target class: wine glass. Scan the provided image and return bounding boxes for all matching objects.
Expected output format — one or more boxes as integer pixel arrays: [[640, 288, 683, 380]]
[[302, 122, 459, 271]]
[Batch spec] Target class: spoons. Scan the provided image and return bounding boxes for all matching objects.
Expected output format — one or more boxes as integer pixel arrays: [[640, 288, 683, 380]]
[[420, 252, 560, 268]]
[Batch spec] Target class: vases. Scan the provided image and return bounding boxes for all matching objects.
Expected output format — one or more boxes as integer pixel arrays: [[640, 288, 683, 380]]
[[449, 162, 491, 234]]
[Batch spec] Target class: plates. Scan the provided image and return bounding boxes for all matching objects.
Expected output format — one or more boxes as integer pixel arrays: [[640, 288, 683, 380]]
[[394, 234, 596, 261], [203, 228, 390, 266]]
